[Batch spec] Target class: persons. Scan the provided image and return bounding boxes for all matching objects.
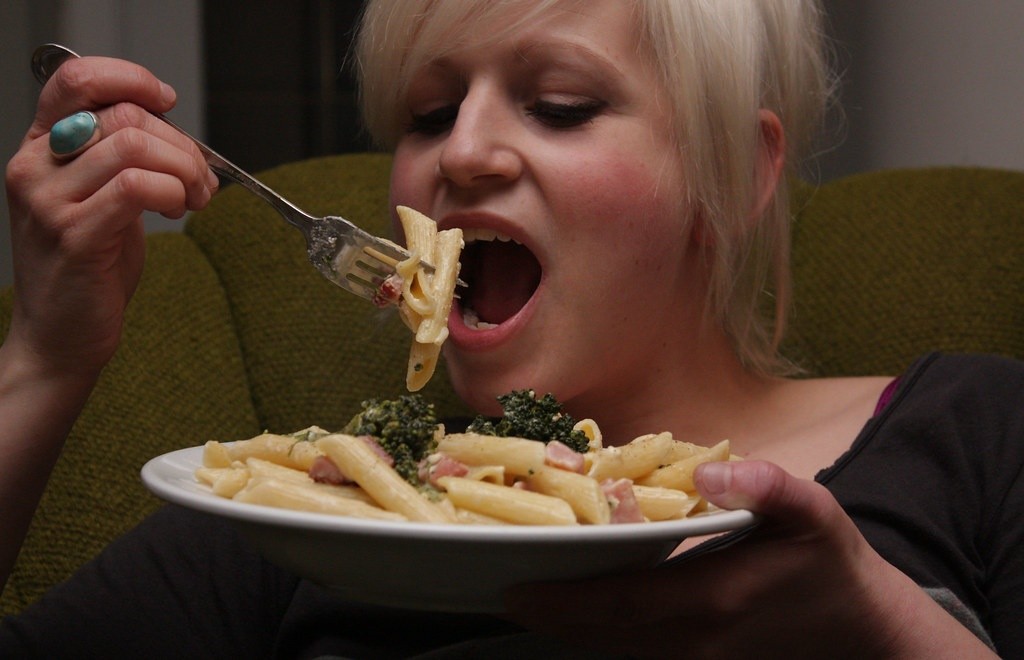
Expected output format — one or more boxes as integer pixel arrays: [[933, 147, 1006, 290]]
[[0, 0, 1024, 660]]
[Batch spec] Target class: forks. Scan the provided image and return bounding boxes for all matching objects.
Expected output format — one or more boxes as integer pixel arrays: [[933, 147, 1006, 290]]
[[31, 44, 467, 310]]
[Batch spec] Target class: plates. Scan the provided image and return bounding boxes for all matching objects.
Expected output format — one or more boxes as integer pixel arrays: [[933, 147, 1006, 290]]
[[141, 442, 755, 539]]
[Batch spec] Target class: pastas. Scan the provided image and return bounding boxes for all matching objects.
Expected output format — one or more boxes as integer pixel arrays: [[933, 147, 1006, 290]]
[[197, 423, 742, 525], [397, 204, 465, 392]]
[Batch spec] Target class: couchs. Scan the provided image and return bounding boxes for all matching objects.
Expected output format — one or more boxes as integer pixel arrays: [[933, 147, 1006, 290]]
[[0, 151, 1024, 660]]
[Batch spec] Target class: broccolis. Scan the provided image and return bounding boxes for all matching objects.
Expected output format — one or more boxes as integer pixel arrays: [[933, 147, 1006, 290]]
[[334, 387, 592, 484]]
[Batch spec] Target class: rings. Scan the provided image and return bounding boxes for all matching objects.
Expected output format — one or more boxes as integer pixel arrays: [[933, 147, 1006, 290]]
[[47, 110, 100, 158]]
